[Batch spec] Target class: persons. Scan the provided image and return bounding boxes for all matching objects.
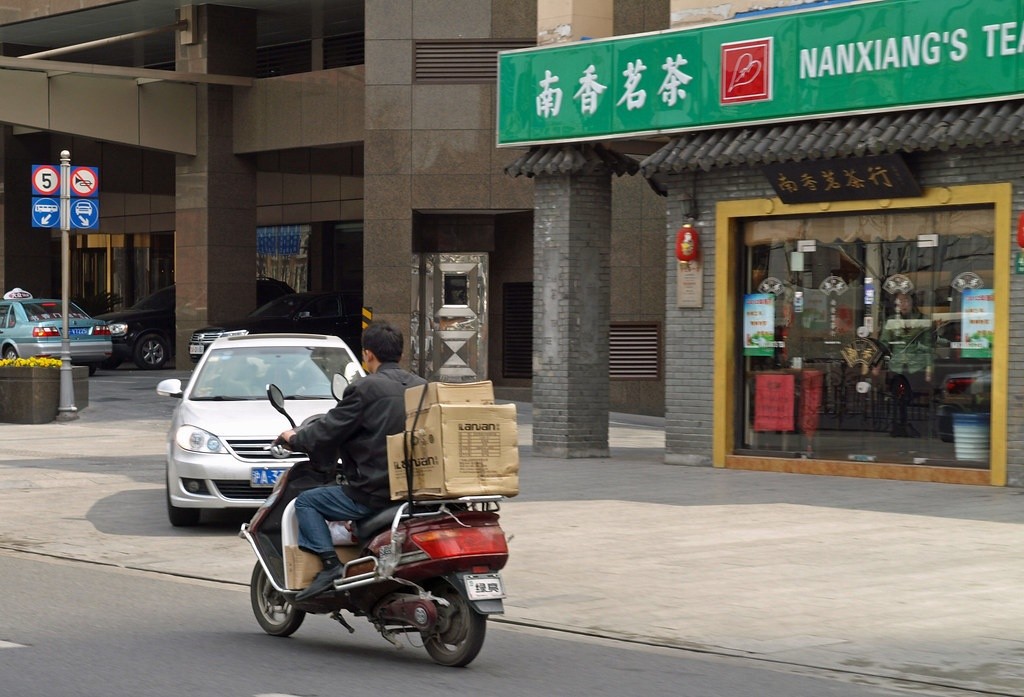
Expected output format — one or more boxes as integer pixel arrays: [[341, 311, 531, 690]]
[[878, 291, 935, 436], [275, 320, 429, 602]]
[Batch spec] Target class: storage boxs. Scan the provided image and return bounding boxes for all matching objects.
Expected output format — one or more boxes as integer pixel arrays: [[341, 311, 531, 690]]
[[386, 380, 521, 502]]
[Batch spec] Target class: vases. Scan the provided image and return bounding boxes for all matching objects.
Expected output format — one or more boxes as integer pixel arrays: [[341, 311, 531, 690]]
[[0, 365, 88, 424]]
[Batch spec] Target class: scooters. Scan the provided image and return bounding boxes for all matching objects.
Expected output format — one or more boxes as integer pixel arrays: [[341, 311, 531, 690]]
[[239, 374, 510, 668]]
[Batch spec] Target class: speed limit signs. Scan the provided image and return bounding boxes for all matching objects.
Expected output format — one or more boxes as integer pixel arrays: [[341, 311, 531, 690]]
[[31, 163, 61, 197]]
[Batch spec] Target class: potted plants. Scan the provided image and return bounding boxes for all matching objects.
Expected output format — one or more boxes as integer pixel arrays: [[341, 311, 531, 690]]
[[945, 389, 991, 462]]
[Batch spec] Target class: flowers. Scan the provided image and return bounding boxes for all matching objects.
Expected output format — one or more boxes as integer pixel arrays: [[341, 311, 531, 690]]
[[0, 355, 61, 367]]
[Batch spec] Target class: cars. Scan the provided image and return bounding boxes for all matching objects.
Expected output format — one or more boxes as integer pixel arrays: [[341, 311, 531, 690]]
[[0, 287, 114, 376], [155, 331, 370, 525], [876, 312, 992, 439], [94, 275, 300, 369], [188, 283, 363, 361]]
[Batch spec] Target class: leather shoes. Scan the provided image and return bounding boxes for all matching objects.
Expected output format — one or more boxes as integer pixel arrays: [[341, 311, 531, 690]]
[[294, 562, 345, 601]]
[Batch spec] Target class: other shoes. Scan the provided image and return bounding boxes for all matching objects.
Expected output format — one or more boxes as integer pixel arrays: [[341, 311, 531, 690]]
[[890, 422, 905, 437], [903, 424, 921, 437]]
